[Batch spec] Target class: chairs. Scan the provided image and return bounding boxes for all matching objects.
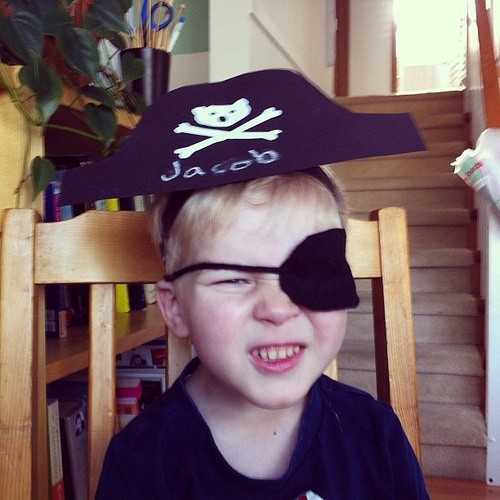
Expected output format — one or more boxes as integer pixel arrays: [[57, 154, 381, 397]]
[[1, 199, 424, 499]]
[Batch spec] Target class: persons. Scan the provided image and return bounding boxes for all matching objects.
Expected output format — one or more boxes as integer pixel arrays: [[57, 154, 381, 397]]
[[56, 70, 445, 500]]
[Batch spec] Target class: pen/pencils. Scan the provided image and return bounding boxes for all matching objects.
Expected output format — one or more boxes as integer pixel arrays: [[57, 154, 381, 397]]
[[112, 0, 187, 53]]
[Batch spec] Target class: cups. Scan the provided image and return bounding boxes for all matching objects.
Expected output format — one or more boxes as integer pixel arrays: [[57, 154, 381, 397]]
[[117, 47, 170, 114]]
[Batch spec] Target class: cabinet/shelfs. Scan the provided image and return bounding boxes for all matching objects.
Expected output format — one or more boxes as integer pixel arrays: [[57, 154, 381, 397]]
[[0, 57, 206, 499]]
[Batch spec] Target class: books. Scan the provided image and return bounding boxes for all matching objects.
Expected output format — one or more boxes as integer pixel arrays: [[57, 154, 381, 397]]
[[28, 151, 166, 339], [54, 400, 93, 500], [57, 328, 186, 429], [41, 396, 64, 500]]
